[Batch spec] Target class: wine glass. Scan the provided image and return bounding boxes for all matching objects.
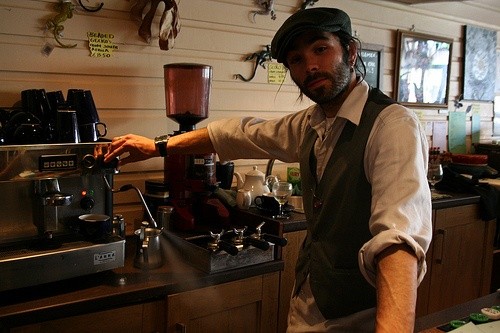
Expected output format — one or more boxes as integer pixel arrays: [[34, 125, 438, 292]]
[[427, 162, 444, 194], [272, 181, 293, 220]]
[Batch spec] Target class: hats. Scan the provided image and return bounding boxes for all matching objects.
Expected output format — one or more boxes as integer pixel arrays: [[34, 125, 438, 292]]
[[271, 7, 351, 63]]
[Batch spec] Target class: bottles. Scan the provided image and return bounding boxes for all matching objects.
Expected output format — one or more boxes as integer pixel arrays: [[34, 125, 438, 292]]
[[113, 214, 125, 239], [156, 206, 175, 233]]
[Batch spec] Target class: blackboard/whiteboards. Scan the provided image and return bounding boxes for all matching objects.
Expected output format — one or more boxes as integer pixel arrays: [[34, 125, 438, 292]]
[[355, 42, 384, 91]]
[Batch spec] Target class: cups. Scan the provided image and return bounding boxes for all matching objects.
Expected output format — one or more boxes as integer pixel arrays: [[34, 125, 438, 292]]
[[78, 213, 111, 242], [215, 161, 234, 189], [235, 189, 252, 211], [0, 88, 108, 145], [133, 225, 164, 269]]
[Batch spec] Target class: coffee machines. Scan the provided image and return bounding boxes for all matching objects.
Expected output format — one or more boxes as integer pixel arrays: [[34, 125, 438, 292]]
[[162, 62, 233, 234]]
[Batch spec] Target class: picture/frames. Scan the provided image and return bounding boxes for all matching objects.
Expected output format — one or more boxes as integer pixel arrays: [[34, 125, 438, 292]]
[[356, 43, 385, 92], [393, 29, 454, 108]]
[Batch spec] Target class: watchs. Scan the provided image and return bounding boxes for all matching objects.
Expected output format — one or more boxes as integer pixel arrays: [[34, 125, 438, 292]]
[[154, 135, 169, 157]]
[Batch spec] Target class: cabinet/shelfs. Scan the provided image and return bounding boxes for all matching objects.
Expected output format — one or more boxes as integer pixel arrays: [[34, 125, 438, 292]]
[[415, 203, 486, 317], [8, 271, 280, 333]]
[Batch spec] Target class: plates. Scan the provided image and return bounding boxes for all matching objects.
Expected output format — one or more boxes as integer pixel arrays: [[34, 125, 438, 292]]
[[469, 304, 500, 322], [449, 319, 466, 328]]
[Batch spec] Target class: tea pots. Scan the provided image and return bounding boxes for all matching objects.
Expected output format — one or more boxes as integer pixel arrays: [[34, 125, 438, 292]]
[[231, 165, 279, 208]]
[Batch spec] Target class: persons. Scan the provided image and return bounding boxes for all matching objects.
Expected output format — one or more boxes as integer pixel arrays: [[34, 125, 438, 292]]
[[103, 6, 433, 333]]
